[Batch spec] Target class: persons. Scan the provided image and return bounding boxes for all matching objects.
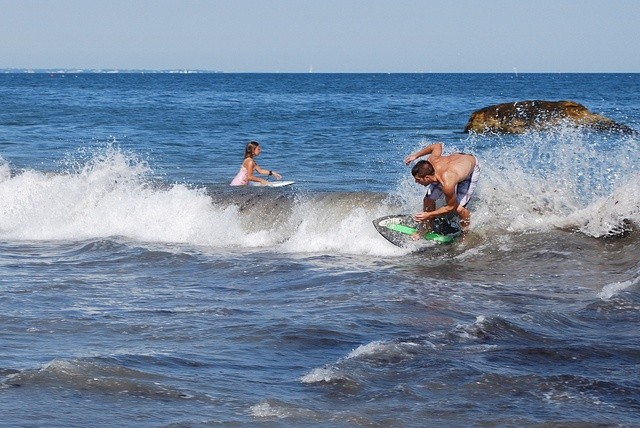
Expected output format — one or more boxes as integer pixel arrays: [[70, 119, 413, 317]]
[[230, 141, 281, 185], [403, 143, 481, 242]]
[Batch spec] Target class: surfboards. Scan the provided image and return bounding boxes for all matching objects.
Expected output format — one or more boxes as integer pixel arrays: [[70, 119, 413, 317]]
[[372, 213, 463, 250], [248, 178, 296, 189]]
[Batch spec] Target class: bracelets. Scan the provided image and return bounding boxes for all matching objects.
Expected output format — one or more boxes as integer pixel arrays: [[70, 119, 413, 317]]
[[269, 171, 272, 175]]
